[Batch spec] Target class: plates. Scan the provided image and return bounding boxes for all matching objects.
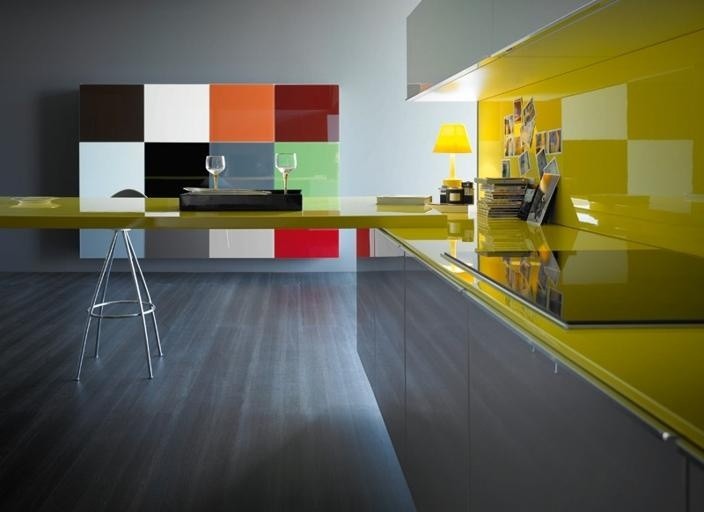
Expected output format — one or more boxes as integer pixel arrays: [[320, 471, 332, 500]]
[[11, 197, 54, 203], [11, 203, 56, 207], [426, 203, 468, 213]]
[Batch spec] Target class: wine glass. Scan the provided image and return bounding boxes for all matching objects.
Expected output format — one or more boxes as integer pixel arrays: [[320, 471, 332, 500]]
[[273, 152, 297, 195], [206, 155, 226, 190]]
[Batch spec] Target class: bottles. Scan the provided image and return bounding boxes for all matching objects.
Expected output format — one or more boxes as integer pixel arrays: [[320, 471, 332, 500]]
[[448, 220, 475, 242], [440, 182, 474, 205]]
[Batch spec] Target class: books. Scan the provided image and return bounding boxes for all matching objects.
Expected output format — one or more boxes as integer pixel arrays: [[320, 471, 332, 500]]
[[377, 195, 433, 205], [477, 218, 528, 258], [474, 175, 528, 218], [378, 205, 431, 212]]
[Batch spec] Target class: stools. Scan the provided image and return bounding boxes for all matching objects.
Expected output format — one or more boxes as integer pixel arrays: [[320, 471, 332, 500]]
[[75, 190, 163, 383]]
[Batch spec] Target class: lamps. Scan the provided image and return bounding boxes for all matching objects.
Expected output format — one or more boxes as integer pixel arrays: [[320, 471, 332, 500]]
[[432, 123, 473, 188]]
